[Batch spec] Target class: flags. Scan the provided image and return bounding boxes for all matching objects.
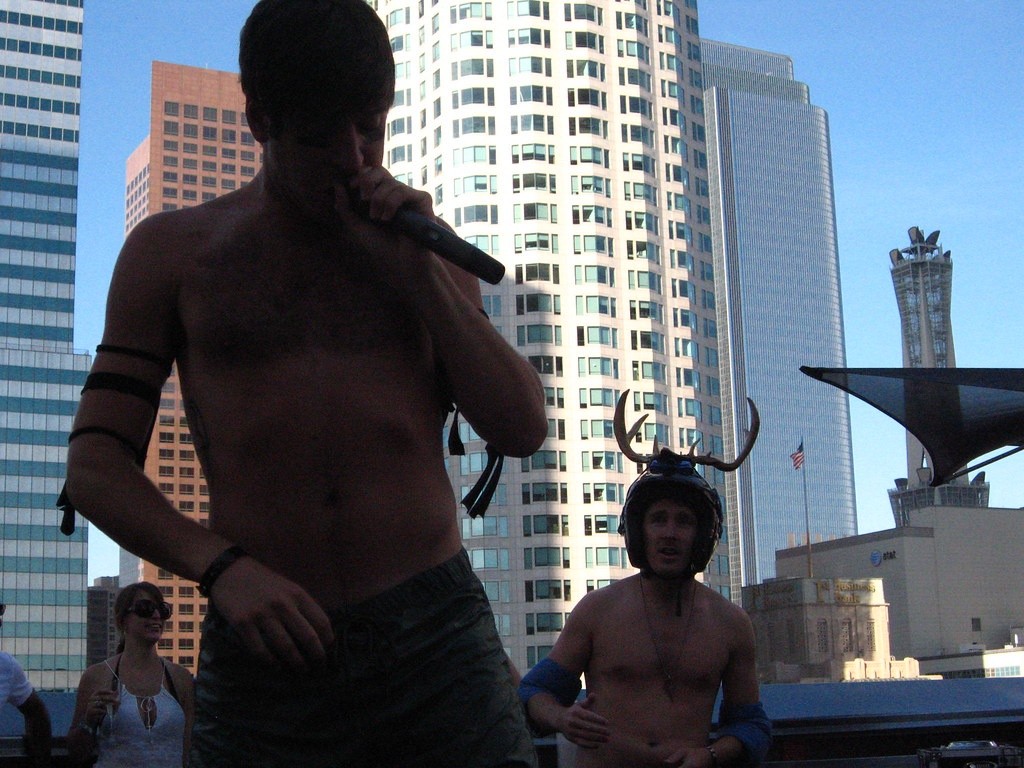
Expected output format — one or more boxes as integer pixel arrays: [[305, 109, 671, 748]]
[[790, 442, 805, 470]]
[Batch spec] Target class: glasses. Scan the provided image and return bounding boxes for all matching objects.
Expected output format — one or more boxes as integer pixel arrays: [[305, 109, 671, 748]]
[[127, 599, 173, 619]]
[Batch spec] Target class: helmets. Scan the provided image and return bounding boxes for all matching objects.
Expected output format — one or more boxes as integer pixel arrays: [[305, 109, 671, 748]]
[[613, 390, 760, 585]]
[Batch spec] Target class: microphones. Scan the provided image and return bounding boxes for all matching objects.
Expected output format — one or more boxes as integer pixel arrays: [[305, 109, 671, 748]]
[[347, 184, 506, 285]]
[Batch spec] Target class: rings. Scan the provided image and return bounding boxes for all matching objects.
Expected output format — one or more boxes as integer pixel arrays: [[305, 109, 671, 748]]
[[95, 701, 102, 706]]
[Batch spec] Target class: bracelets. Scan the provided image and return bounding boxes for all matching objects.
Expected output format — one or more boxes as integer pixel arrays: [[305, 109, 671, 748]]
[[79, 722, 93, 735], [706, 746, 717, 768], [196, 545, 247, 596]]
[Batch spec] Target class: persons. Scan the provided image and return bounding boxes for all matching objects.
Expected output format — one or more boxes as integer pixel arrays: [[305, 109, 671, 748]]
[[519, 448, 771, 768], [0, 603, 52, 768], [66, 582, 196, 768], [62, 0, 547, 768]]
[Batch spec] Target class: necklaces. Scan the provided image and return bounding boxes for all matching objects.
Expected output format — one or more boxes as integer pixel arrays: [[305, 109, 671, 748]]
[[640, 575, 697, 688]]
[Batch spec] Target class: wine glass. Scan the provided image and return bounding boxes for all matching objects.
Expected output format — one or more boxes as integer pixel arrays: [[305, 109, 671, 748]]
[[103, 679, 122, 745]]
